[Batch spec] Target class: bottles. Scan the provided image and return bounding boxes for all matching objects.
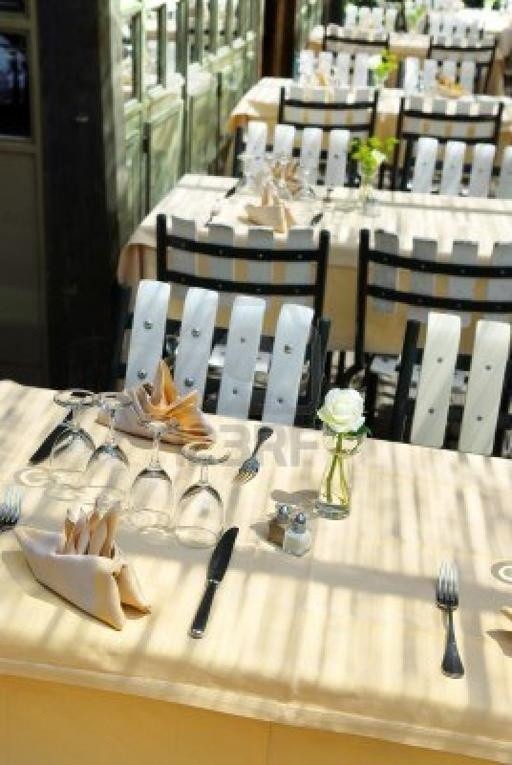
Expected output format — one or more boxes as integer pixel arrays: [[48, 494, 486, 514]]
[[265, 504, 312, 557]]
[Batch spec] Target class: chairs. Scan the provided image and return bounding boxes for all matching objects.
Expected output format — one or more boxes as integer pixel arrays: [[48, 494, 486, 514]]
[[355, 227, 510, 456], [299, 2, 510, 96], [278, 88, 379, 185], [390, 97, 506, 189], [154, 213, 331, 429], [230, 119, 359, 192], [388, 311, 506, 458], [122, 277, 325, 430], [400, 138, 510, 199]]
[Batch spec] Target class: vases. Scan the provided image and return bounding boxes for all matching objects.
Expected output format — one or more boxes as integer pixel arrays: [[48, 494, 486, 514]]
[[358, 176, 382, 218], [314, 423, 368, 520]]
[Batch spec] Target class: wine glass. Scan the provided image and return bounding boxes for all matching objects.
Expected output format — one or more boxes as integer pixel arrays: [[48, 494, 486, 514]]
[[50, 390, 230, 549], [235, 151, 323, 227]]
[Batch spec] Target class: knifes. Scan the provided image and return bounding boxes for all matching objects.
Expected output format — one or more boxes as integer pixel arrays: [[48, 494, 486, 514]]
[[188, 526, 240, 640], [29, 395, 72, 467]]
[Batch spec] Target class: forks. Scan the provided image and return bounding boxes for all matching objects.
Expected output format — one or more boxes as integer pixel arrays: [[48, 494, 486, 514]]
[[233, 426, 273, 484], [0, 492, 23, 537], [432, 560, 471, 677]]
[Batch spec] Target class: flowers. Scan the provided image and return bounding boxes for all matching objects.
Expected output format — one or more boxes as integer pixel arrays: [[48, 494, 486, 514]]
[[314, 387, 373, 505], [348, 134, 402, 183]]
